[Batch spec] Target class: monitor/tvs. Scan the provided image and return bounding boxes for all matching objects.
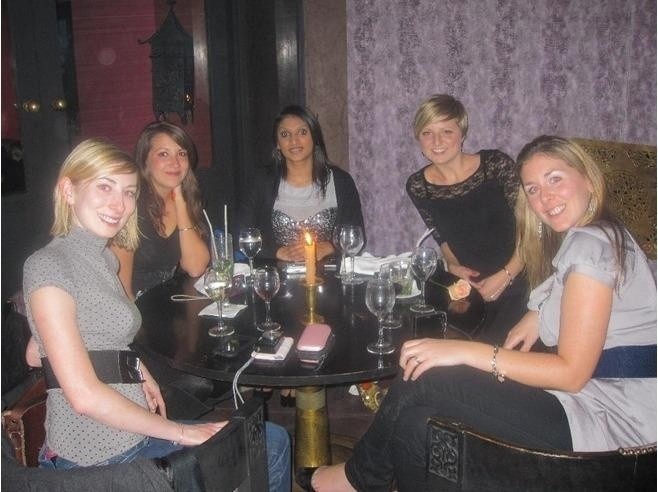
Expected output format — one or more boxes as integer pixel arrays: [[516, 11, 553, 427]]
[[254, 328, 285, 355]]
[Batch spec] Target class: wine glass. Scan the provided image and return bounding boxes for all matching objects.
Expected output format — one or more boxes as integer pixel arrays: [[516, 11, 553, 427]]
[[238, 228, 262, 283], [409, 246, 437, 313], [331, 225, 351, 278], [204, 267, 235, 337], [364, 279, 395, 354], [340, 225, 364, 286], [211, 233, 233, 275], [378, 264, 402, 328], [252, 266, 281, 333]]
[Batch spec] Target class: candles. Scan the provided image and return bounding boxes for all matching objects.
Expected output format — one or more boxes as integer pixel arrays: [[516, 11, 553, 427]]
[[304, 232, 316, 284]]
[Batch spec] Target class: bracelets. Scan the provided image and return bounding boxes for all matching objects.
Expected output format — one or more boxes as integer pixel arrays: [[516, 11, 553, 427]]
[[503, 263, 514, 288], [493, 343, 504, 386], [175, 420, 185, 447], [176, 226, 197, 233]]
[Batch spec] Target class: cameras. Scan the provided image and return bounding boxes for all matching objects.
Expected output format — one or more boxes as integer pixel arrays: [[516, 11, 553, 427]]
[[286, 262, 306, 274], [224, 273, 247, 298], [412, 310, 448, 339]]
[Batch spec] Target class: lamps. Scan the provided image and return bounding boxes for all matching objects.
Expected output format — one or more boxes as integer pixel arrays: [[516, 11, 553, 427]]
[[135, 1, 196, 127]]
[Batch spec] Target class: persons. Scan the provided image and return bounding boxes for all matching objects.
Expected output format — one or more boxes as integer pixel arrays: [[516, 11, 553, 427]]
[[134, 275, 204, 365], [113, 119, 210, 301], [22, 136, 291, 490], [238, 107, 366, 406], [404, 92, 537, 345], [297, 136, 655, 491]]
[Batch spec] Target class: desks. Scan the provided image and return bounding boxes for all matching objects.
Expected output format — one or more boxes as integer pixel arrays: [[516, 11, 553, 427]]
[[126, 255, 481, 492]]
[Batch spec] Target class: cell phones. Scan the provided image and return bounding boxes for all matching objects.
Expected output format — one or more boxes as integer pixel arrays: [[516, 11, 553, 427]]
[[297, 323, 332, 351], [324, 257, 337, 268]]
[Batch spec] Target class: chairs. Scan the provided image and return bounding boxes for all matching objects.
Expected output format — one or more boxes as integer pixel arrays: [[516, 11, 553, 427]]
[[2, 376, 269, 492], [424, 414, 656, 491]]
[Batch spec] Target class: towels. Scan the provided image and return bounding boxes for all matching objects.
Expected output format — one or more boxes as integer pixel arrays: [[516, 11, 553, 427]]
[[193, 262, 250, 299], [341, 252, 413, 276]]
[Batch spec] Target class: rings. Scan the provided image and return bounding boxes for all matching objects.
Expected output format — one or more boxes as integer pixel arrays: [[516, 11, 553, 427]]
[[415, 357, 421, 366], [216, 426, 222, 433]]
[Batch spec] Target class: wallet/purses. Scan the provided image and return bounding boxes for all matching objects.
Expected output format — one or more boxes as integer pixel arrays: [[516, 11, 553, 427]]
[[250, 336, 294, 360]]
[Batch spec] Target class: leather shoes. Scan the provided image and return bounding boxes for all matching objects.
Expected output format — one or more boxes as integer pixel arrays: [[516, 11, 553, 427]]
[[288, 392, 297, 408], [295, 465, 318, 492], [262, 387, 273, 401], [252, 387, 263, 399], [279, 392, 290, 407], [359, 382, 387, 415]]
